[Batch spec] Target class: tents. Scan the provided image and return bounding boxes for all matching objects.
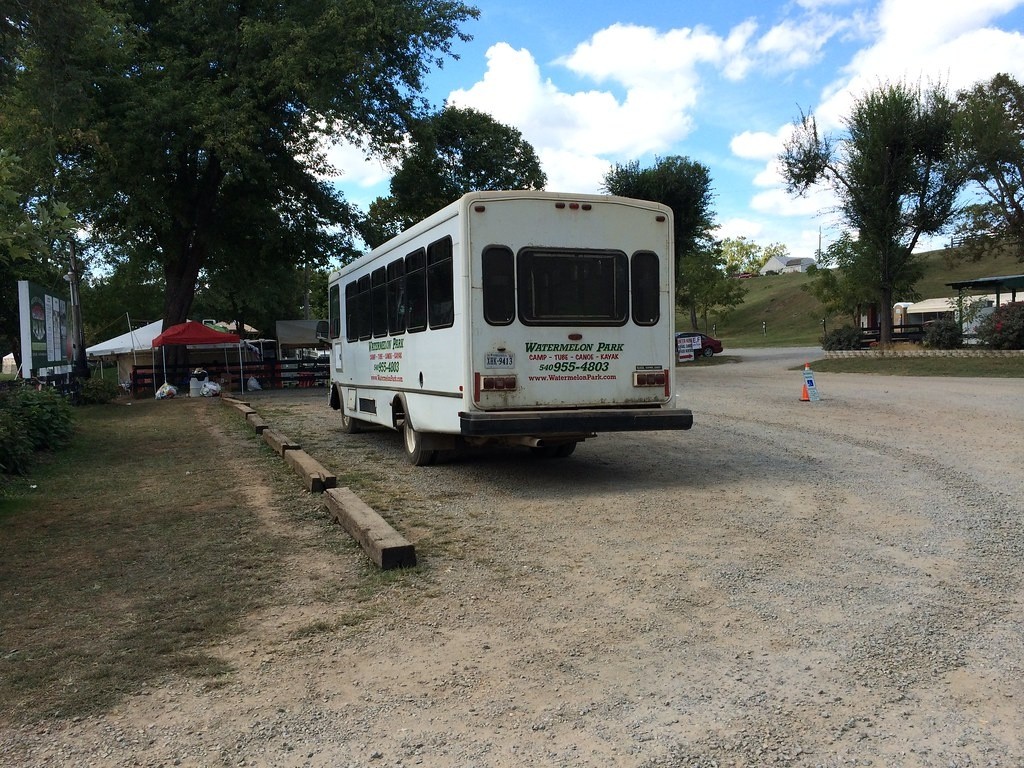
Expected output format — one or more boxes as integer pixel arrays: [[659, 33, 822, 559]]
[[85, 317, 243, 395], [152, 321, 243, 394]]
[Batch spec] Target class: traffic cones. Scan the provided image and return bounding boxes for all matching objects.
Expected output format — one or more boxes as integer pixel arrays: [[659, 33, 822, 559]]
[[798, 362, 811, 402]]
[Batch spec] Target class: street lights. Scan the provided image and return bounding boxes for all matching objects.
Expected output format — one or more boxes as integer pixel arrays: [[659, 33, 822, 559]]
[[258, 338, 265, 362]]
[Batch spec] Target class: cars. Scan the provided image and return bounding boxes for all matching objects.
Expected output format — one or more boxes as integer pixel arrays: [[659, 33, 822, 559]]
[[308, 349, 330, 366], [738, 272, 752, 279], [675, 332, 723, 358]]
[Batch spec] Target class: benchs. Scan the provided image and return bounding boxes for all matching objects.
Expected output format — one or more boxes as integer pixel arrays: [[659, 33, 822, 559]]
[[130, 357, 330, 393]]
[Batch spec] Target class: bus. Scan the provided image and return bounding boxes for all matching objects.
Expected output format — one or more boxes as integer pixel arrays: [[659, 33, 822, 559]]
[[316, 189, 695, 467]]
